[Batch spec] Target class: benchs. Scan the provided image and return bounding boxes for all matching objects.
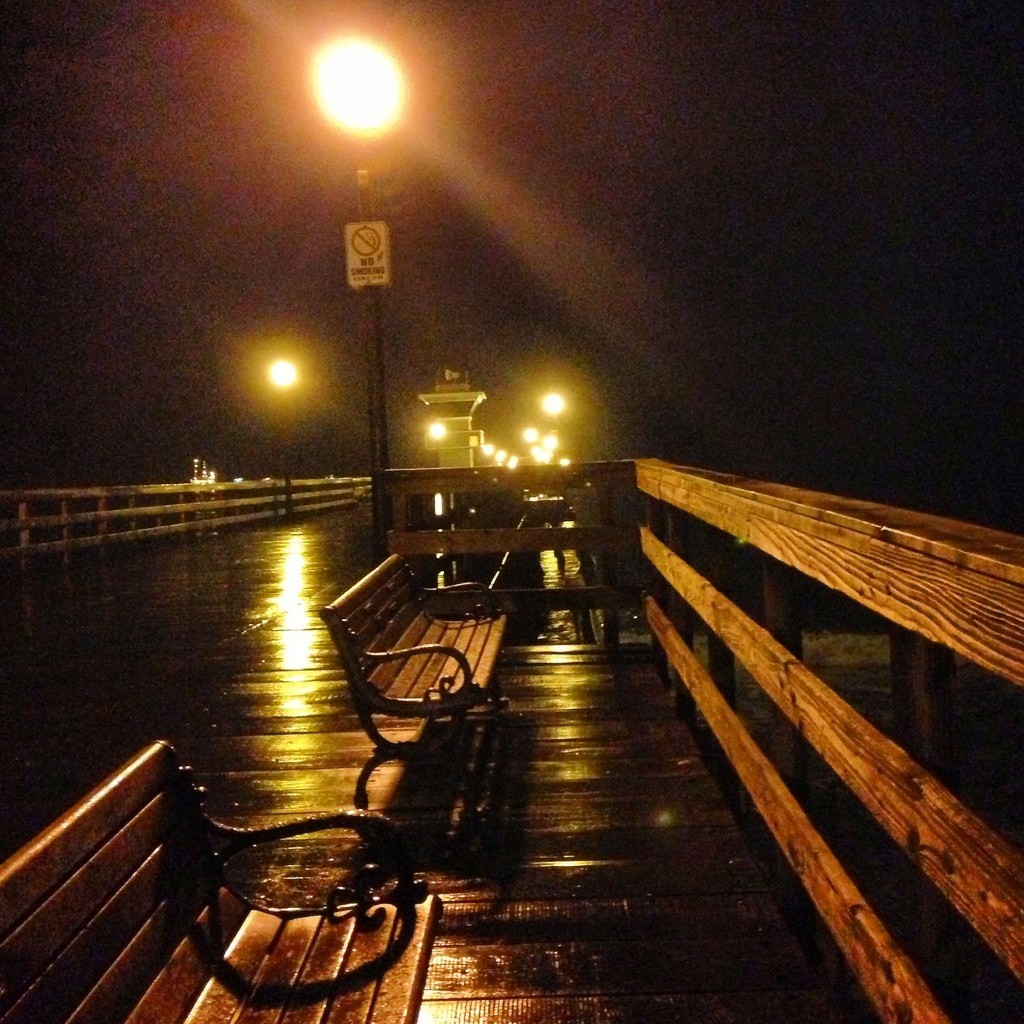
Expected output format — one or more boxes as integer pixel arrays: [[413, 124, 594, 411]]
[[0, 739, 443, 1024], [318, 553, 508, 822]]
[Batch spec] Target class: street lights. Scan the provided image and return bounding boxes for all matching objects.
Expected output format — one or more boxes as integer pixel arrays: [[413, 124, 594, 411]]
[[312, 37, 393, 567], [268, 359, 299, 520]]
